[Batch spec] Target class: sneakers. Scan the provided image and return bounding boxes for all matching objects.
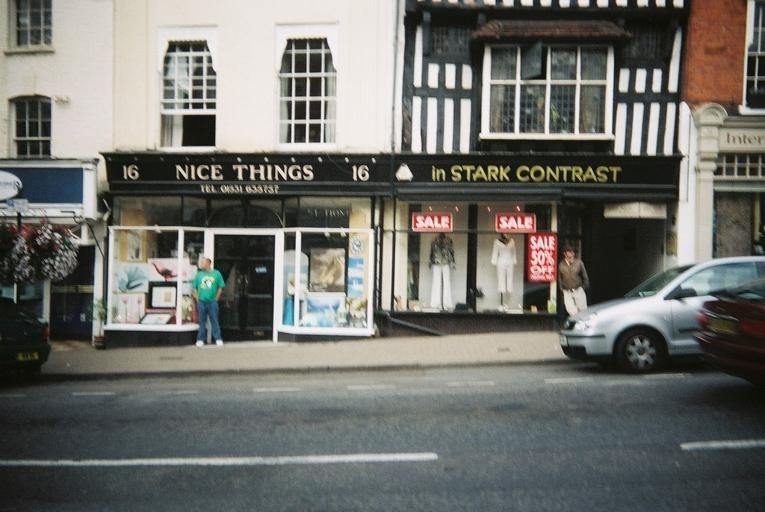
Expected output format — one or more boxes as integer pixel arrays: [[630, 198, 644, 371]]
[[216, 339, 223, 347], [195, 340, 204, 348]]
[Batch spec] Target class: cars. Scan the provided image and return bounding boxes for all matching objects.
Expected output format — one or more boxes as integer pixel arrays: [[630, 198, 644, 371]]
[[559, 256, 765, 373], [0, 296, 50, 375], [692, 276, 765, 390]]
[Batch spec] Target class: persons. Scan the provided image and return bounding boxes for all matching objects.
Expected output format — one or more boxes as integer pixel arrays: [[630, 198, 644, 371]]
[[557, 244, 591, 316], [492, 235, 518, 294], [428, 232, 456, 310], [193, 257, 226, 347]]
